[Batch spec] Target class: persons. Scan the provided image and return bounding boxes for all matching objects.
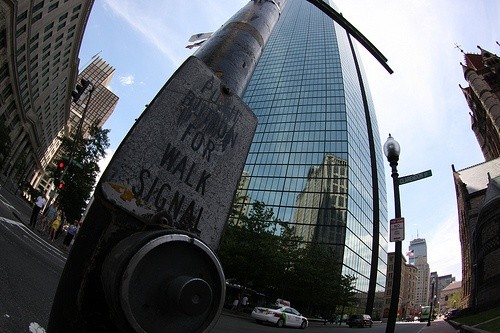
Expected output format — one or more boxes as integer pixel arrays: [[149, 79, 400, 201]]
[[39, 201, 70, 244], [231, 295, 248, 314], [28, 192, 46, 230], [61, 220, 78, 251]]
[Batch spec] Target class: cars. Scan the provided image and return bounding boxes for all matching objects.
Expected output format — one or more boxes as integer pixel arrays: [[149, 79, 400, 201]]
[[252, 304, 308, 329]]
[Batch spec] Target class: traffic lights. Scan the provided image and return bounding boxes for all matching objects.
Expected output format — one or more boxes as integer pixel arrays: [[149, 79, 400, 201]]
[[71, 78, 90, 103], [54, 158, 64, 190]]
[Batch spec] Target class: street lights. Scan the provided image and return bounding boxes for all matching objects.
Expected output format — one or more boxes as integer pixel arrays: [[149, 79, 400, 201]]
[[383, 132, 404, 333]]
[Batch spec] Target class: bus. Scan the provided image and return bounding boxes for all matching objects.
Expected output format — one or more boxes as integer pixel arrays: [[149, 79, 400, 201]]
[[420, 306, 434, 321]]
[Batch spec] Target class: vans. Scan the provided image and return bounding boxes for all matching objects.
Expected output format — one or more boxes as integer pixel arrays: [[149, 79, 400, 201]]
[[348, 314, 373, 328]]
